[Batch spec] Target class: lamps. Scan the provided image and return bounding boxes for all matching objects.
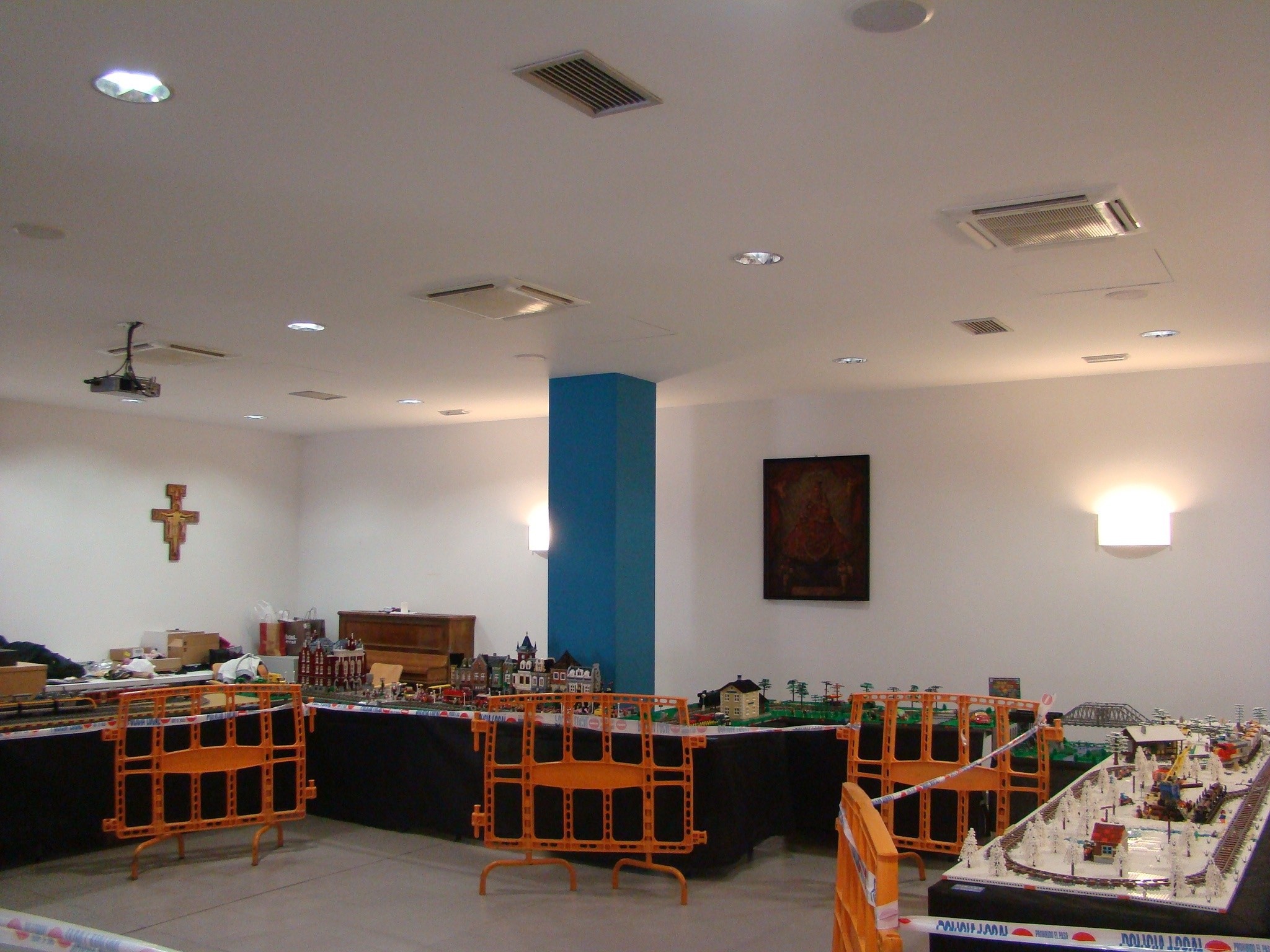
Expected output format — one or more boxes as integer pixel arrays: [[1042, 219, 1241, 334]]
[[1097, 514, 1170, 546]]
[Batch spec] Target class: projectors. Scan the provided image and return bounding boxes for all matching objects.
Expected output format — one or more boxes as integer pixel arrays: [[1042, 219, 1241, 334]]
[[89, 377, 161, 398]]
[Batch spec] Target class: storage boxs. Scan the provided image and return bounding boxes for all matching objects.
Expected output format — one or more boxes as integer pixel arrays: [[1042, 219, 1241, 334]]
[[0, 662, 48, 702], [150, 658, 182, 672], [0, 649, 17, 667], [168, 632, 221, 668], [110, 648, 143, 661]]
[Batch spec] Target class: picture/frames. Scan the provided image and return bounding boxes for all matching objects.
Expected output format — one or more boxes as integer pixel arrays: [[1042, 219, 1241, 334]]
[[762, 454, 870, 600]]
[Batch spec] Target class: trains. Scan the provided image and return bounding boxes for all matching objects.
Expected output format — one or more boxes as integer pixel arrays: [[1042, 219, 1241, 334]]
[[1211, 721, 1261, 766]]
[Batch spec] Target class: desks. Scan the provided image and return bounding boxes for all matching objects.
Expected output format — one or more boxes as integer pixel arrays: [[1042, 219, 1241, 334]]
[[0, 680, 1270, 952]]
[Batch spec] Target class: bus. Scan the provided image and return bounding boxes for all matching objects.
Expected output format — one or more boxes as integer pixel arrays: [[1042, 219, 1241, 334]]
[[443, 687, 473, 705], [427, 684, 452, 700]]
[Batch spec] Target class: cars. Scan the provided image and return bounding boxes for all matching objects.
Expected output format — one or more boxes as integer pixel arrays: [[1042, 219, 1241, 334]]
[[405, 689, 415, 700], [972, 712, 990, 724], [1178, 726, 1191, 735]]
